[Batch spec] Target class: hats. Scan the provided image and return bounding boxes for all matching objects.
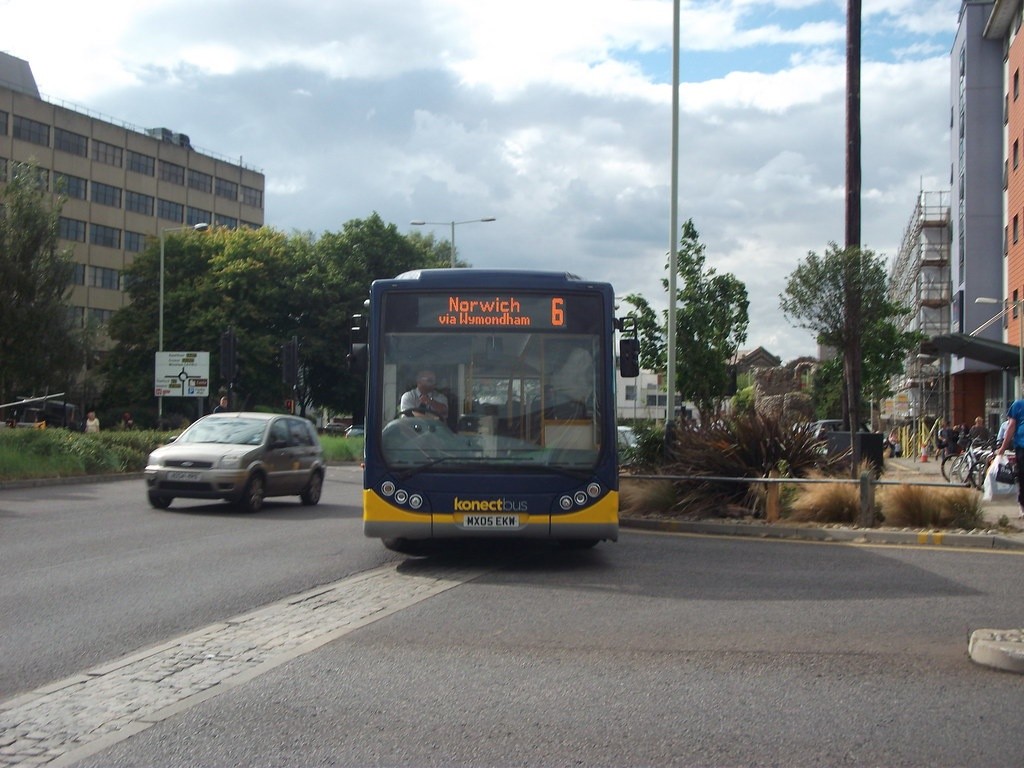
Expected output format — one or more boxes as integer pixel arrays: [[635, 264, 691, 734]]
[[975, 417, 983, 422]]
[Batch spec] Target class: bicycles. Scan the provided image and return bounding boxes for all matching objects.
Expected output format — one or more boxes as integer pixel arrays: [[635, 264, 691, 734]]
[[937, 421, 1018, 498]]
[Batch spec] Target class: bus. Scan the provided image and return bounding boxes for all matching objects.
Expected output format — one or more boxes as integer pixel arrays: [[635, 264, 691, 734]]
[[346, 266, 640, 559]]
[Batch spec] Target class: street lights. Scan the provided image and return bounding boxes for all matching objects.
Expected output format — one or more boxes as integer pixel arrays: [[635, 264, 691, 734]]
[[410, 217, 496, 269], [155, 222, 210, 433]]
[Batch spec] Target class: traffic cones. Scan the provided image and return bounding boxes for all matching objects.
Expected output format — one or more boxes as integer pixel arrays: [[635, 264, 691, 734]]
[[920, 441, 928, 463]]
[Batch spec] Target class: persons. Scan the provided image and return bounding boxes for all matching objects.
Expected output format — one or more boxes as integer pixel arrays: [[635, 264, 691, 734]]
[[887, 428, 901, 457], [998, 383, 1024, 518], [85, 411, 100, 433], [935, 416, 990, 462], [400, 370, 448, 423], [996, 409, 1013, 449], [213, 396, 230, 413]]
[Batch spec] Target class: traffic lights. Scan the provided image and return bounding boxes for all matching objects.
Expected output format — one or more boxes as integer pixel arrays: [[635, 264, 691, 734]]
[[285, 399, 295, 415]]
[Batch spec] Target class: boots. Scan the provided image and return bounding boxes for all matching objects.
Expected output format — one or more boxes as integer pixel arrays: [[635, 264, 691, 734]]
[[1019, 504, 1024, 519]]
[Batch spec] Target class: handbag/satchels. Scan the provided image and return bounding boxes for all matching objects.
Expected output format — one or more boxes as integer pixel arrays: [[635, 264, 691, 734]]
[[935, 429, 950, 450], [984, 453, 1016, 499]]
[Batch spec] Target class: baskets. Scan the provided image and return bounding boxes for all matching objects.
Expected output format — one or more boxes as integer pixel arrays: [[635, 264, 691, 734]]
[[957, 436, 971, 450]]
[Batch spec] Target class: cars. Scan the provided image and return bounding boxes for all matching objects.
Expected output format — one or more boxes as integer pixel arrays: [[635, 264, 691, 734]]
[[317, 422, 364, 440], [805, 419, 871, 462]]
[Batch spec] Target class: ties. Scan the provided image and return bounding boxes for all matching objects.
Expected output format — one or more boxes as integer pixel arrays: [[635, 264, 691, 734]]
[[418, 394, 428, 408]]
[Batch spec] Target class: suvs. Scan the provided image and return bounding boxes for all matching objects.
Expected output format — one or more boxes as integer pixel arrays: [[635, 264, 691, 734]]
[[142, 412, 327, 513]]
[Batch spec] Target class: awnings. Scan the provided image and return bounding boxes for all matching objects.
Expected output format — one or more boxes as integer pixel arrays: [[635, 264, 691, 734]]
[[917, 333, 1024, 379]]
